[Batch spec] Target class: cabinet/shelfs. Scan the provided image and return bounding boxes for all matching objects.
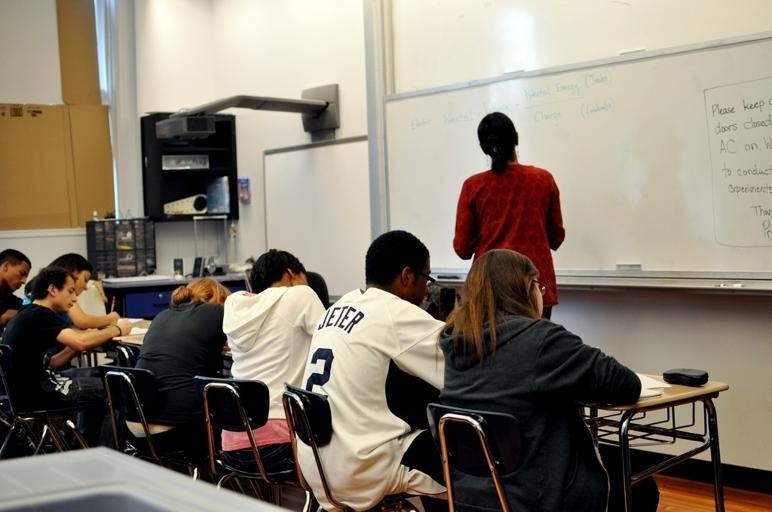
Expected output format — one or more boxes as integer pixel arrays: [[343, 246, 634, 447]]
[[140, 115, 239, 220]]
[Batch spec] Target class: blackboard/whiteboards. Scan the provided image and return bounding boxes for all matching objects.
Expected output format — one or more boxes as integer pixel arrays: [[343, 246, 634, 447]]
[[263, 135, 373, 300], [384, 28, 772, 282]]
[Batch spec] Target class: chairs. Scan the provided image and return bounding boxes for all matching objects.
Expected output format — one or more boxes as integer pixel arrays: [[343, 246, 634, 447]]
[[281, 382, 415, 512], [425, 400, 528, 512], [0, 345, 88, 456], [193, 376, 300, 500], [96, 366, 182, 465]]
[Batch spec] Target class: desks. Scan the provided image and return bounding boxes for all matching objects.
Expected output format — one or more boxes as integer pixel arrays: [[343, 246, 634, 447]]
[[103, 273, 249, 325], [583, 375, 731, 510]]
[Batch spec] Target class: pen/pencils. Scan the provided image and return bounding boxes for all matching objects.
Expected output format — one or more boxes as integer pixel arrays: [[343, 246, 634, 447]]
[[111, 296, 116, 311]]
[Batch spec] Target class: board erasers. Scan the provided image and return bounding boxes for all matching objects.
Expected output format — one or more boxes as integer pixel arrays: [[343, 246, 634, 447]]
[[616, 265, 641, 271]]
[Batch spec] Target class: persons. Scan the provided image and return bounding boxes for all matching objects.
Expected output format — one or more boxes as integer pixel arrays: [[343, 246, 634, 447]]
[[297, 230, 448, 511], [0, 248, 129, 448], [119, 277, 232, 462], [450, 110, 566, 322], [438, 249, 659, 510], [222, 250, 327, 479]]
